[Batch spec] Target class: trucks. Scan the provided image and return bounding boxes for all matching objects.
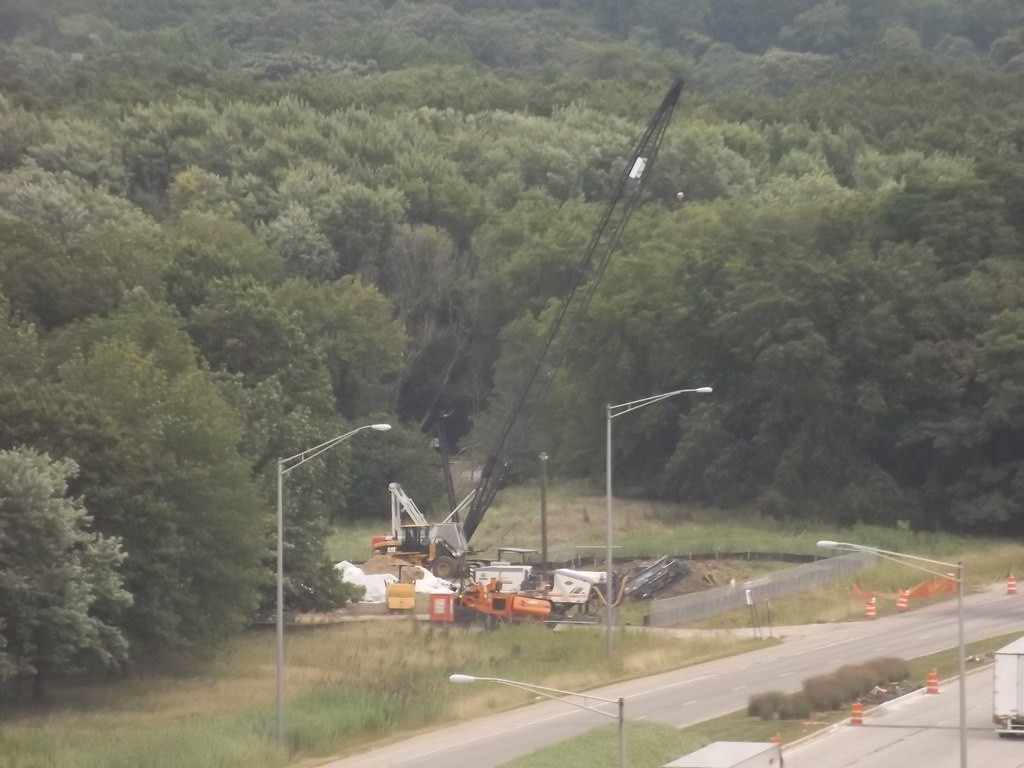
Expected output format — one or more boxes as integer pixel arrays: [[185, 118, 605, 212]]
[[992, 636, 1024, 738]]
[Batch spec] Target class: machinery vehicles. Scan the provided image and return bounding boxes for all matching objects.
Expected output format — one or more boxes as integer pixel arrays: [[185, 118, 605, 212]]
[[371, 78, 691, 631]]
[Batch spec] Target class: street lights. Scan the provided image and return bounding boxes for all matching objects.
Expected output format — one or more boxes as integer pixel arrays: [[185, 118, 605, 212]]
[[449, 674, 625, 768], [815, 541, 966, 768], [276, 424, 393, 743], [606, 387, 712, 656]]
